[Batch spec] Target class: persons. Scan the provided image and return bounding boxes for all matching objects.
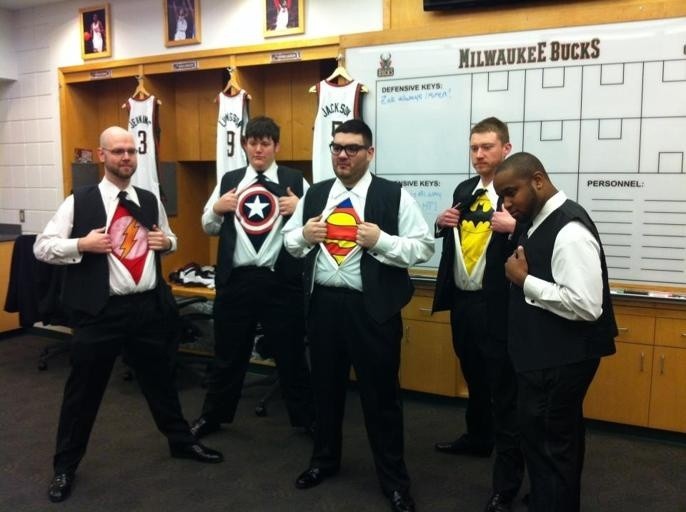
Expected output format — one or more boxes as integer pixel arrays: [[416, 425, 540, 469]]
[[429, 115, 528, 511], [191, 115, 312, 441], [280, 118, 437, 512], [32, 125, 224, 502], [491, 153, 620, 512]]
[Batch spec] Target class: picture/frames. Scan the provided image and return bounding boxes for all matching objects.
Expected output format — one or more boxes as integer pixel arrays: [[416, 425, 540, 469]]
[[77, 2, 114, 60], [160, 0, 203, 48], [260, 0, 307, 39]]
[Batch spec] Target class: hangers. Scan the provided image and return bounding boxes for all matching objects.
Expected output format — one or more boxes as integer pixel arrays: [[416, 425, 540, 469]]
[[120, 74, 163, 110], [307, 55, 370, 94], [211, 69, 254, 103]]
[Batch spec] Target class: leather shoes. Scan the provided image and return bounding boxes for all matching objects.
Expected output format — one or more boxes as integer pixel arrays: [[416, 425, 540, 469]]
[[295, 465, 338, 489], [169, 442, 223, 464], [521, 492, 531, 504], [484, 490, 512, 512], [303, 420, 316, 441], [47, 471, 75, 502], [190, 417, 220, 437], [389, 490, 415, 512], [435, 440, 462, 455]]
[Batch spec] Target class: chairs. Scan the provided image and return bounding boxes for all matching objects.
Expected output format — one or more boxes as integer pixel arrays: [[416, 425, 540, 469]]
[[9, 232, 118, 372]]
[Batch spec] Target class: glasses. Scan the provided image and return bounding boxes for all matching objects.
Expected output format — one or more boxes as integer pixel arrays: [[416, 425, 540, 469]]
[[103, 147, 138, 155], [328, 141, 364, 158]]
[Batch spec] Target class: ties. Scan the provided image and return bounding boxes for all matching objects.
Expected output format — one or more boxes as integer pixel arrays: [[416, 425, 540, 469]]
[[118, 190, 152, 228], [258, 173, 285, 198], [458, 187, 486, 214]]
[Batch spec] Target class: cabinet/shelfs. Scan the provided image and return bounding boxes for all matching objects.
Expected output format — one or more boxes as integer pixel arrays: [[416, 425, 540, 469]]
[[48, 36, 352, 363], [1, 235, 22, 333], [395, 285, 470, 399], [581, 295, 686, 433]]
